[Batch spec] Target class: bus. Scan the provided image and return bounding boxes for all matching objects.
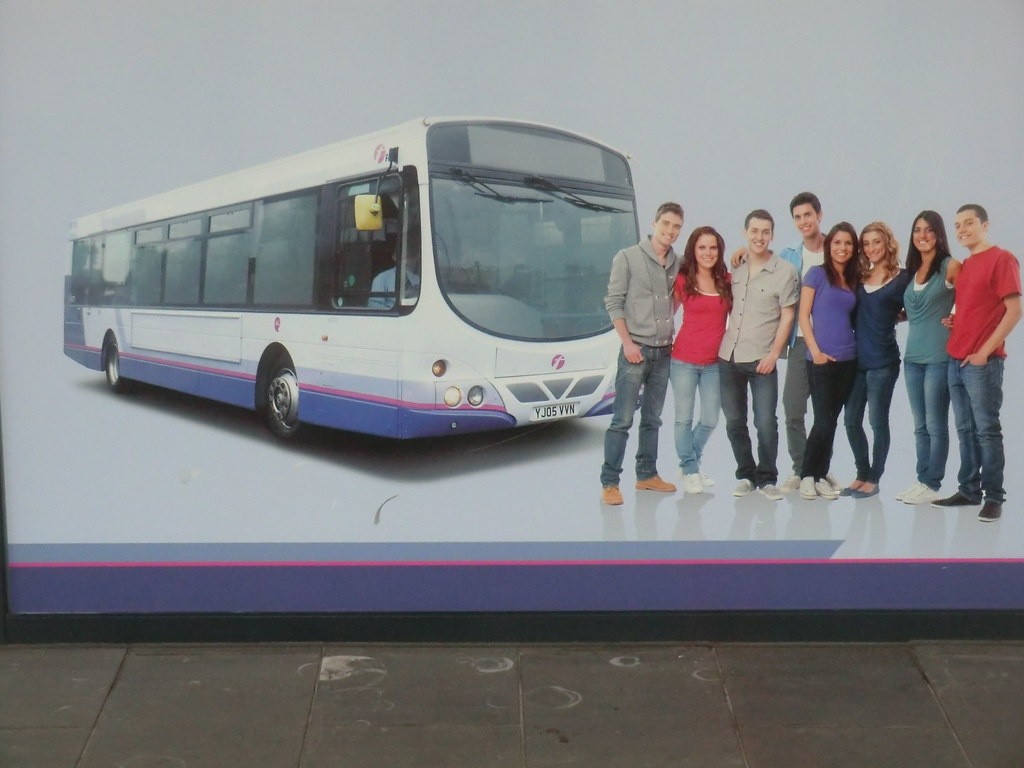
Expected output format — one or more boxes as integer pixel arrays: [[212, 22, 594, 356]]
[[63, 117, 646, 447]]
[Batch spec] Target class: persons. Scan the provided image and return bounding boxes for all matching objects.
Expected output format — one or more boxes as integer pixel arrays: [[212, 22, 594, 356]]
[[731, 191, 842, 494], [368, 243, 421, 309], [896, 209, 962, 505], [931, 203, 1023, 522], [600, 201, 685, 507], [840, 220, 909, 499], [719, 208, 799, 500], [672, 225, 732, 494], [798, 222, 858, 503]]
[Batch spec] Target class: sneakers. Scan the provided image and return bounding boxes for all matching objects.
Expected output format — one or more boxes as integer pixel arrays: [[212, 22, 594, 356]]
[[760, 484, 783, 500], [700, 472, 715, 487], [684, 473, 703, 493], [903, 483, 939, 504], [734, 478, 756, 496], [895, 482, 922, 501]]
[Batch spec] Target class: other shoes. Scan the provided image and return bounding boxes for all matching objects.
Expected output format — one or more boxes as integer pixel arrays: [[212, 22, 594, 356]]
[[815, 482, 838, 500], [839, 486, 862, 496], [800, 480, 816, 499], [603, 486, 623, 505], [636, 473, 677, 492], [826, 475, 843, 495], [780, 476, 801, 491], [852, 484, 879, 498], [929, 492, 981, 508], [977, 501, 1002, 521]]
